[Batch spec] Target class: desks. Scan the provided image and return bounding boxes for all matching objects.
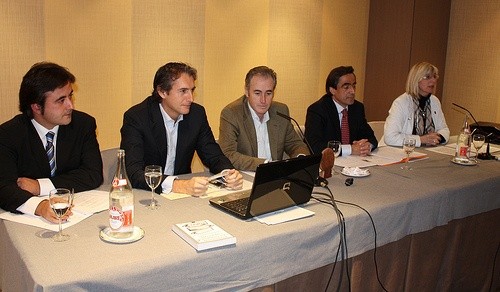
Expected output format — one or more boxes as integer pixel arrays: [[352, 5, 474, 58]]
[[0, 137, 500, 292]]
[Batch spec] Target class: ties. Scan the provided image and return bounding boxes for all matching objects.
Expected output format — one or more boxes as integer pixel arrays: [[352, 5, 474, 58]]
[[340, 109, 351, 144], [44, 131, 57, 178]]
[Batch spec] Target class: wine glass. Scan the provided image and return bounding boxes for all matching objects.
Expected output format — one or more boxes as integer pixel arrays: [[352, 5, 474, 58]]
[[401, 136, 416, 171], [48, 189, 72, 242], [144, 165, 163, 212], [457, 133, 471, 157], [471, 135, 486, 164], [327, 140, 342, 175]]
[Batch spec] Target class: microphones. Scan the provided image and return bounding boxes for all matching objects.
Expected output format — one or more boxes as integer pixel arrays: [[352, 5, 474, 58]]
[[276, 111, 328, 187], [451, 102, 491, 143]]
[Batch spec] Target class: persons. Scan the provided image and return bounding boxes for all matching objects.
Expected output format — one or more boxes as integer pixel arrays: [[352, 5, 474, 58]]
[[0, 63, 104, 224], [121, 62, 244, 196], [377, 62, 450, 147], [219, 66, 310, 171], [304, 66, 378, 157]]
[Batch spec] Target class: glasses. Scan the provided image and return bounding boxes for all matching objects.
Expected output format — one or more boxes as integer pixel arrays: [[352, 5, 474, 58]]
[[422, 73, 438, 80]]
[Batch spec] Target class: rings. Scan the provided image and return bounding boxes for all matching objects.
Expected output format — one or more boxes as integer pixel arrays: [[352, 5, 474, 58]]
[[434, 139, 435, 142]]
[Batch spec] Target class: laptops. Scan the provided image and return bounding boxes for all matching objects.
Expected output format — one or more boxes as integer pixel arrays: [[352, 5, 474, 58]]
[[209, 152, 322, 220]]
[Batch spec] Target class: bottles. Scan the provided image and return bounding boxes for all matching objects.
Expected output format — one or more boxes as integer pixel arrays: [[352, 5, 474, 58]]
[[455, 114, 470, 158], [107, 150, 135, 237]]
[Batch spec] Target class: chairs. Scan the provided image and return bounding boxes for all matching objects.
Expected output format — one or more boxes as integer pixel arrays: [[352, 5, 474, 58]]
[[100, 147, 120, 183], [366, 120, 387, 141], [282, 126, 306, 159]]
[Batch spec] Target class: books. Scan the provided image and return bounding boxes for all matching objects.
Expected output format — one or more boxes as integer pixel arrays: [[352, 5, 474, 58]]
[[173, 219, 236, 251]]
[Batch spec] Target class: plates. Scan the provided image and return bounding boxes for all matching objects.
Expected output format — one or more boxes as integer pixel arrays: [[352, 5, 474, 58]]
[[342, 166, 372, 177], [451, 158, 477, 166], [100, 226, 145, 244]]
